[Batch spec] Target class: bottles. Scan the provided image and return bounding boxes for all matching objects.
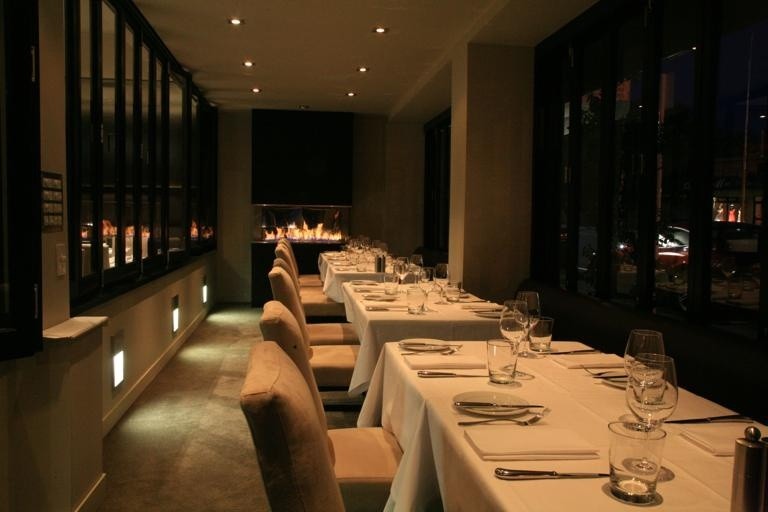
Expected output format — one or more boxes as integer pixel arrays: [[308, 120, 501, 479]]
[[375, 254, 385, 273]]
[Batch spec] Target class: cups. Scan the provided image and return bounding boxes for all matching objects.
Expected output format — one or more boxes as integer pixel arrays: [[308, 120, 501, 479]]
[[406, 288, 425, 316], [384, 274, 399, 296], [608, 422, 668, 503], [487, 339, 519, 385], [448, 284, 461, 303], [529, 318, 555, 354]]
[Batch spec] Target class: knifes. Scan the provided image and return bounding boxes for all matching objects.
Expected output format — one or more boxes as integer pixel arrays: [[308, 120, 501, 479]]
[[456, 400, 545, 409], [495, 467, 611, 480], [664, 413, 751, 426], [416, 370, 488, 378], [548, 347, 599, 355]]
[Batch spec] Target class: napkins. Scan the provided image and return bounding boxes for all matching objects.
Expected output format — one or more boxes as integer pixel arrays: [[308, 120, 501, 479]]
[[361, 302, 409, 308], [404, 355, 487, 370], [679, 425, 768, 456], [460, 302, 502, 309], [351, 285, 385, 290], [545, 354, 631, 369], [464, 425, 601, 462]]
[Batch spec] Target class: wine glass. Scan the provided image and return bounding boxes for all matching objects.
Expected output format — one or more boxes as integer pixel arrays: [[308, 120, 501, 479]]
[[623, 328, 678, 474], [435, 263, 452, 306], [396, 254, 425, 296], [502, 292, 542, 359]]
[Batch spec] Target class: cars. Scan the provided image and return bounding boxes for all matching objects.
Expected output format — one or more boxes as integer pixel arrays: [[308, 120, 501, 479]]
[[615, 224, 723, 284]]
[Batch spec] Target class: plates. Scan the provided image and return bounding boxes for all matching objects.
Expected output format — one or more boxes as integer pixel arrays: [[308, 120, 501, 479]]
[[602, 369, 629, 388], [397, 336, 452, 352], [453, 391, 530, 417]]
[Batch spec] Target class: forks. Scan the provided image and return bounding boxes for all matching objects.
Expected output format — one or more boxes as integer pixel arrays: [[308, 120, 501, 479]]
[[459, 416, 543, 427]]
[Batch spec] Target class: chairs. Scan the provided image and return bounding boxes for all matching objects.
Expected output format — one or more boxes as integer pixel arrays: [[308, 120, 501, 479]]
[[258, 237, 368, 395], [240, 341, 404, 512]]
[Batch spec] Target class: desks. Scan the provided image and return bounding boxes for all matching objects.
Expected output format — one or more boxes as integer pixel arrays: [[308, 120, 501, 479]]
[[356, 339, 768, 511], [341, 281, 528, 398], [318, 250, 426, 303]]
